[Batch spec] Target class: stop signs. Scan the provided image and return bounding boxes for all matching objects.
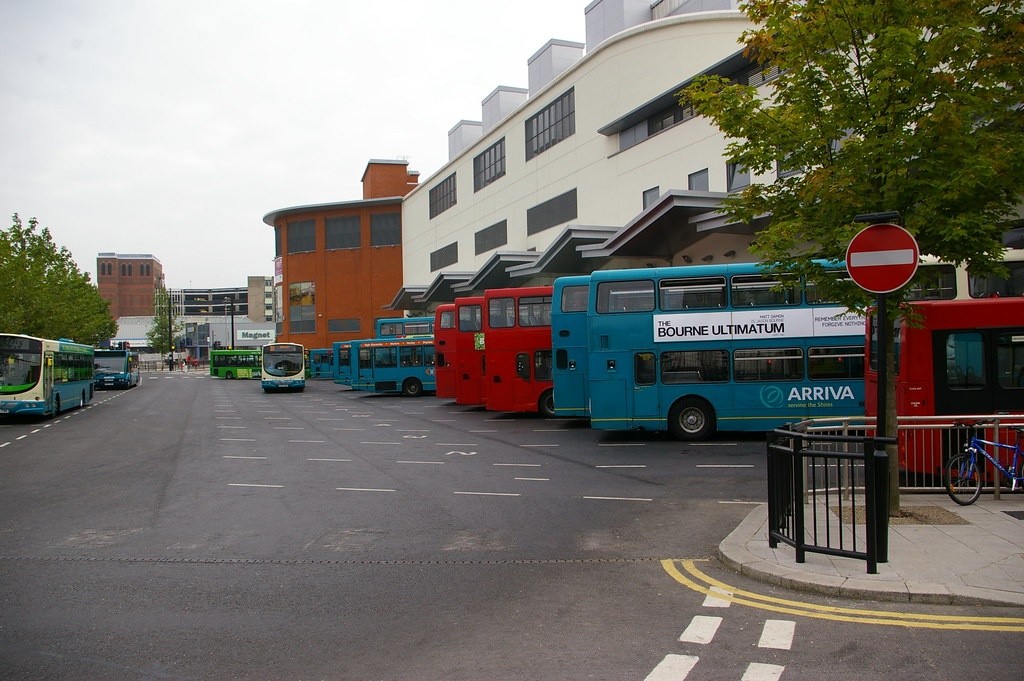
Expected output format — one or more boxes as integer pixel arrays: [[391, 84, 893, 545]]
[[845, 222, 920, 294]]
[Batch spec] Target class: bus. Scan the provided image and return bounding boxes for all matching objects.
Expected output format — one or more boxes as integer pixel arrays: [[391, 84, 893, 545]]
[[586, 245, 1022, 443], [0, 333, 96, 419], [261, 343, 311, 393], [348, 335, 434, 399], [92, 349, 140, 389], [481, 285, 554, 418], [209, 349, 313, 381], [433, 304, 454, 399], [550, 275, 800, 418], [453, 296, 485, 407], [861, 295, 1024, 483], [375, 316, 481, 339], [310, 338, 372, 385]]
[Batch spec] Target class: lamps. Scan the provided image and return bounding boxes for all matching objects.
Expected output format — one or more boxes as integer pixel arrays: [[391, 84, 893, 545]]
[[647, 263, 656, 267], [682, 255, 692, 264], [747, 239, 756, 246], [701, 255, 714, 261], [724, 250, 735, 257]]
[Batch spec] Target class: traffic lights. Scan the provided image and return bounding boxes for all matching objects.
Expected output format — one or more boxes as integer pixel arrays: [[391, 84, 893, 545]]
[[126, 341, 131, 349], [117, 342, 123, 350]]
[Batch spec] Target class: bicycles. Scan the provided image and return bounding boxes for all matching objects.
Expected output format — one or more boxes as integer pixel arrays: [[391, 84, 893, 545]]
[[943, 420, 1024, 506]]
